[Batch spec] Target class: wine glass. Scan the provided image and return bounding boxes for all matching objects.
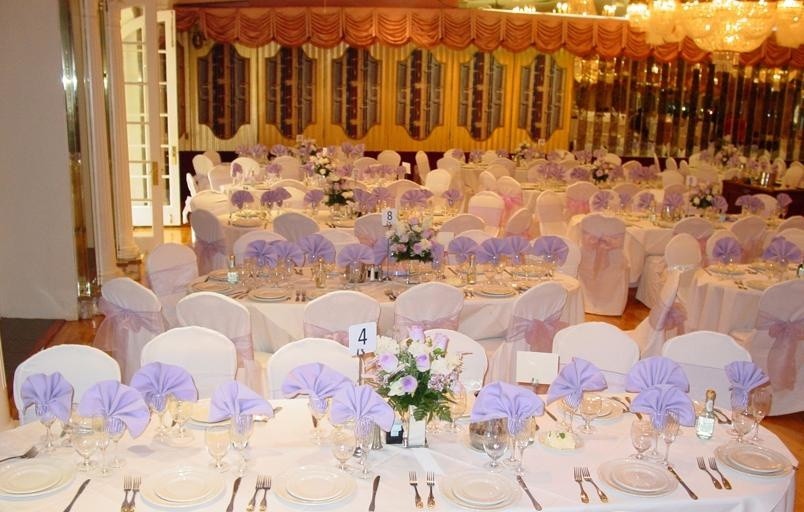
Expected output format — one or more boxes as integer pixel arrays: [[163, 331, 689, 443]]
[[768, 259, 789, 286], [721, 256, 735, 280], [240, 256, 559, 287], [0, 389, 770, 476]]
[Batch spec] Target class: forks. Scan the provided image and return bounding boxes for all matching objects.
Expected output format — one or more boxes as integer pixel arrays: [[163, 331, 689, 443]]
[[247, 472, 264, 512], [572, 465, 590, 504], [425, 471, 436, 510], [128, 472, 143, 511], [695, 456, 722, 489], [122, 474, 132, 512], [259, 471, 272, 511], [580, 465, 608, 503], [1, 445, 38, 463], [708, 456, 733, 490], [409, 471, 424, 509]]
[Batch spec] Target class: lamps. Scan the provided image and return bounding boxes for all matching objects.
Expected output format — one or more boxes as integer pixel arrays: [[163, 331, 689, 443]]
[[626, 0, 804, 71]]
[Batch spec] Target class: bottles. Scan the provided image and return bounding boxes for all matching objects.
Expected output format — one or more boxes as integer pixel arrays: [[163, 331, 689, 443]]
[[695, 390, 716, 441]]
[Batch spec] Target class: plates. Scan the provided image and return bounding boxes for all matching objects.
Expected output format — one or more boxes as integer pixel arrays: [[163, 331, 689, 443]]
[[0, 443, 793, 509], [602, 398, 623, 420], [191, 398, 229, 423], [597, 400, 612, 418], [253, 289, 288, 299], [482, 286, 514, 295], [247, 291, 291, 303], [473, 287, 516, 297]]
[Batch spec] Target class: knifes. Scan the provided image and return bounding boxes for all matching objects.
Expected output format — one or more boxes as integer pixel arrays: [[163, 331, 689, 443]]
[[63, 478, 92, 512], [369, 476, 380, 511], [226, 476, 243, 512], [664, 461, 698, 501], [516, 475, 542, 510]]
